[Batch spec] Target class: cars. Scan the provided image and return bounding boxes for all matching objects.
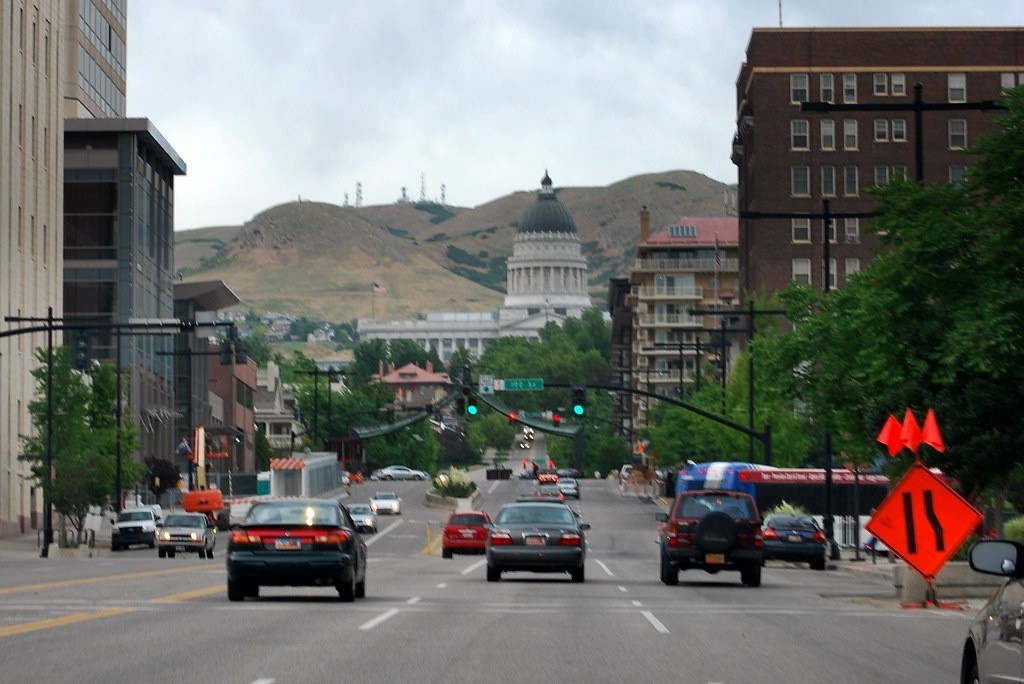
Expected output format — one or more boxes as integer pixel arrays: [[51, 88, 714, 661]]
[[346, 503, 379, 533], [959, 539, 1024, 684], [369, 491, 403, 515], [226, 500, 367, 603], [761, 513, 827, 570], [158, 511, 217, 559], [485, 468, 592, 583], [370, 464, 431, 481], [218, 503, 253, 531]]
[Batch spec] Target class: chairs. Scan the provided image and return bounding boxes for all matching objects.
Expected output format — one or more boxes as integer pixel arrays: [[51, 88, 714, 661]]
[[262, 509, 282, 522]]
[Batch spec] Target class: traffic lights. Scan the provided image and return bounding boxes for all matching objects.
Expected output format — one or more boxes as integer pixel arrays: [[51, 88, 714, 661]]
[[571, 385, 586, 419], [468, 397, 478, 417], [553, 413, 560, 428], [457, 398, 466, 417]]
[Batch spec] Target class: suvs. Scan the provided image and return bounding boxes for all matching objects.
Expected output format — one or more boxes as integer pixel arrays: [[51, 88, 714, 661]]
[[619, 464, 633, 483], [655, 490, 759, 585], [442, 510, 496, 560], [111, 507, 163, 550]]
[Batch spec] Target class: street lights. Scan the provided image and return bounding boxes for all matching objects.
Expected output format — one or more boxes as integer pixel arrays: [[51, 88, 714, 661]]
[[641, 82, 1012, 555], [6, 313, 174, 557]]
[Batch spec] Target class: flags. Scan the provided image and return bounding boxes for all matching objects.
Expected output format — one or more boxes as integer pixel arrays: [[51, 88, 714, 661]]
[[877, 408, 944, 457]]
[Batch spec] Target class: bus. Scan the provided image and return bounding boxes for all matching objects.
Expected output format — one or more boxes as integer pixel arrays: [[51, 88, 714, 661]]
[[673, 461, 946, 559]]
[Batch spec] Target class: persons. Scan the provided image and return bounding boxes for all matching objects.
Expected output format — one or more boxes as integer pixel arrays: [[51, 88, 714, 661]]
[[532, 463, 539, 479]]
